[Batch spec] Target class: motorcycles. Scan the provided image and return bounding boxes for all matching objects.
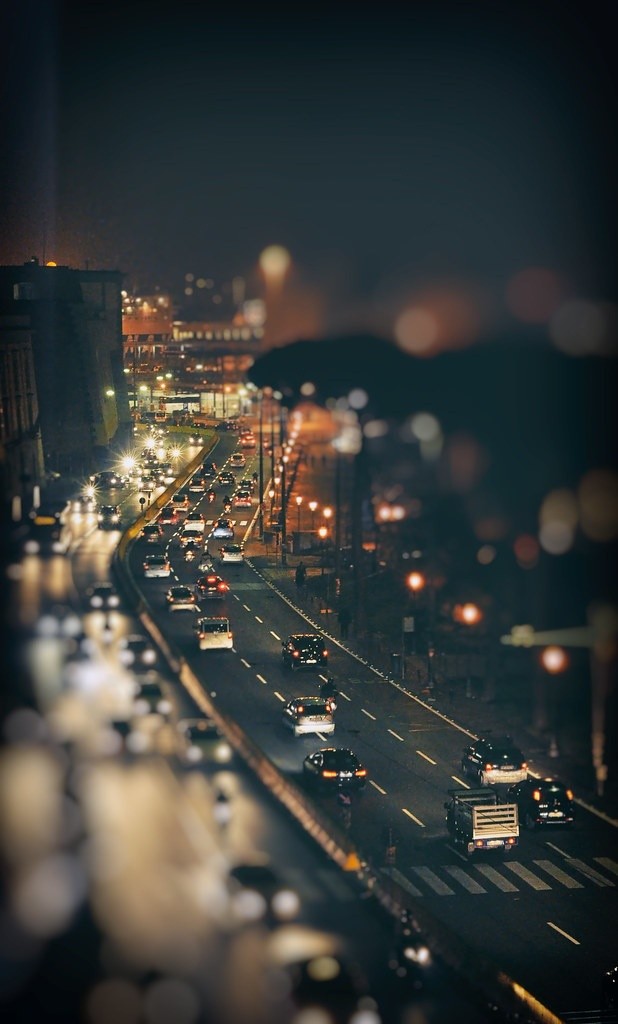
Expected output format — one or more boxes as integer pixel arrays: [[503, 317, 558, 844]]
[[197, 557, 216, 575], [318, 685, 338, 716], [183, 544, 196, 563]]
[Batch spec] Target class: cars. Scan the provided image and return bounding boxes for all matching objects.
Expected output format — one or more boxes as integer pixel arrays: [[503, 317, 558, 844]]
[[506, 777, 577, 830], [156, 507, 180, 526], [282, 696, 335, 738], [169, 492, 191, 512], [140, 524, 165, 545], [218, 543, 244, 566], [197, 574, 227, 600], [211, 518, 234, 540], [89, 409, 258, 513], [303, 747, 368, 794], [182, 513, 208, 534], [179, 529, 204, 549], [164, 585, 196, 613], [142, 553, 171, 578]]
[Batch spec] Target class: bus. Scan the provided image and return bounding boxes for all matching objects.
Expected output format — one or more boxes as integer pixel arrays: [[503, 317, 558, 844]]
[[157, 393, 200, 415]]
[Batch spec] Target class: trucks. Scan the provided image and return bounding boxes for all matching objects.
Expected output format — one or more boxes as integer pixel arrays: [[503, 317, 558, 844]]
[[443, 787, 520, 864]]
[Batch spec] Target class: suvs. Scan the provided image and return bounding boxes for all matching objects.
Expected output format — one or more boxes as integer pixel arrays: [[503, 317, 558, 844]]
[[280, 634, 329, 673], [459, 736, 529, 790]]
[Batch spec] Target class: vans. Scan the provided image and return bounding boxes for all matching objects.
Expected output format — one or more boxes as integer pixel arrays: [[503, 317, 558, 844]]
[[192, 617, 233, 652]]
